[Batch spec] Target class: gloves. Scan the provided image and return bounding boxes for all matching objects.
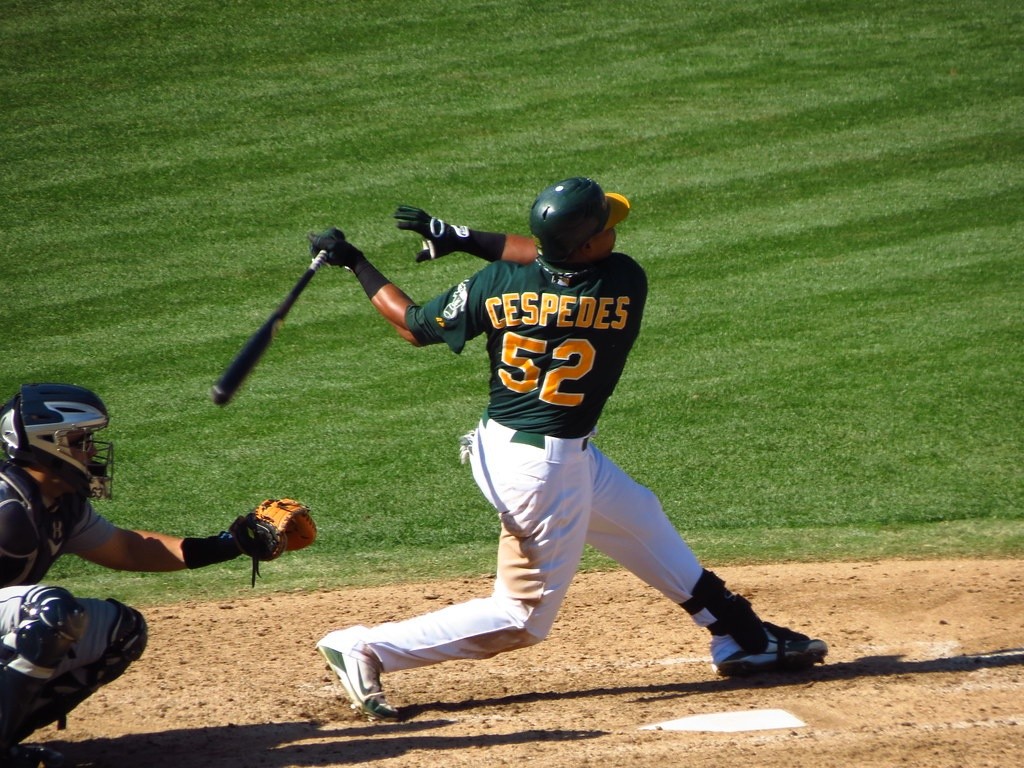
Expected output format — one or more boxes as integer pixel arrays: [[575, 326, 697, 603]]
[[307, 229, 392, 298], [395, 204, 507, 262]]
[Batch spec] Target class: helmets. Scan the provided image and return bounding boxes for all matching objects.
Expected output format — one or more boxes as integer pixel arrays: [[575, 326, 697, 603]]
[[0, 382, 108, 489], [530, 176, 629, 262]]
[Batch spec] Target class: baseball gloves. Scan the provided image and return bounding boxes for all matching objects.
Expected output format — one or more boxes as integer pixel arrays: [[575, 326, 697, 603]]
[[235, 496, 317, 560]]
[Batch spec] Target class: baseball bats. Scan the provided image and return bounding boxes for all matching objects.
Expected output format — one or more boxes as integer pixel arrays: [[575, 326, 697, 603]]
[[210, 226, 346, 408]]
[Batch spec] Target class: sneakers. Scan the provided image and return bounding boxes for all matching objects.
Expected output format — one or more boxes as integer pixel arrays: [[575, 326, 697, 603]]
[[711, 621, 828, 677], [315, 626, 399, 721]]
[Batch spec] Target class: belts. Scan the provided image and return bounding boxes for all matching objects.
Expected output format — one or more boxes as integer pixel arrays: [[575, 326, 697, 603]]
[[482, 407, 590, 453]]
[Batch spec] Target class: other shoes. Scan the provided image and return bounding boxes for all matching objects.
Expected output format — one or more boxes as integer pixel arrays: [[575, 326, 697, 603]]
[[2, 744, 64, 768]]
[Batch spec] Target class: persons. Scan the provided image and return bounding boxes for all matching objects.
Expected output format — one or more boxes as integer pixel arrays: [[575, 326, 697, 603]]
[[314, 176, 828, 720], [0, 381, 318, 767]]
